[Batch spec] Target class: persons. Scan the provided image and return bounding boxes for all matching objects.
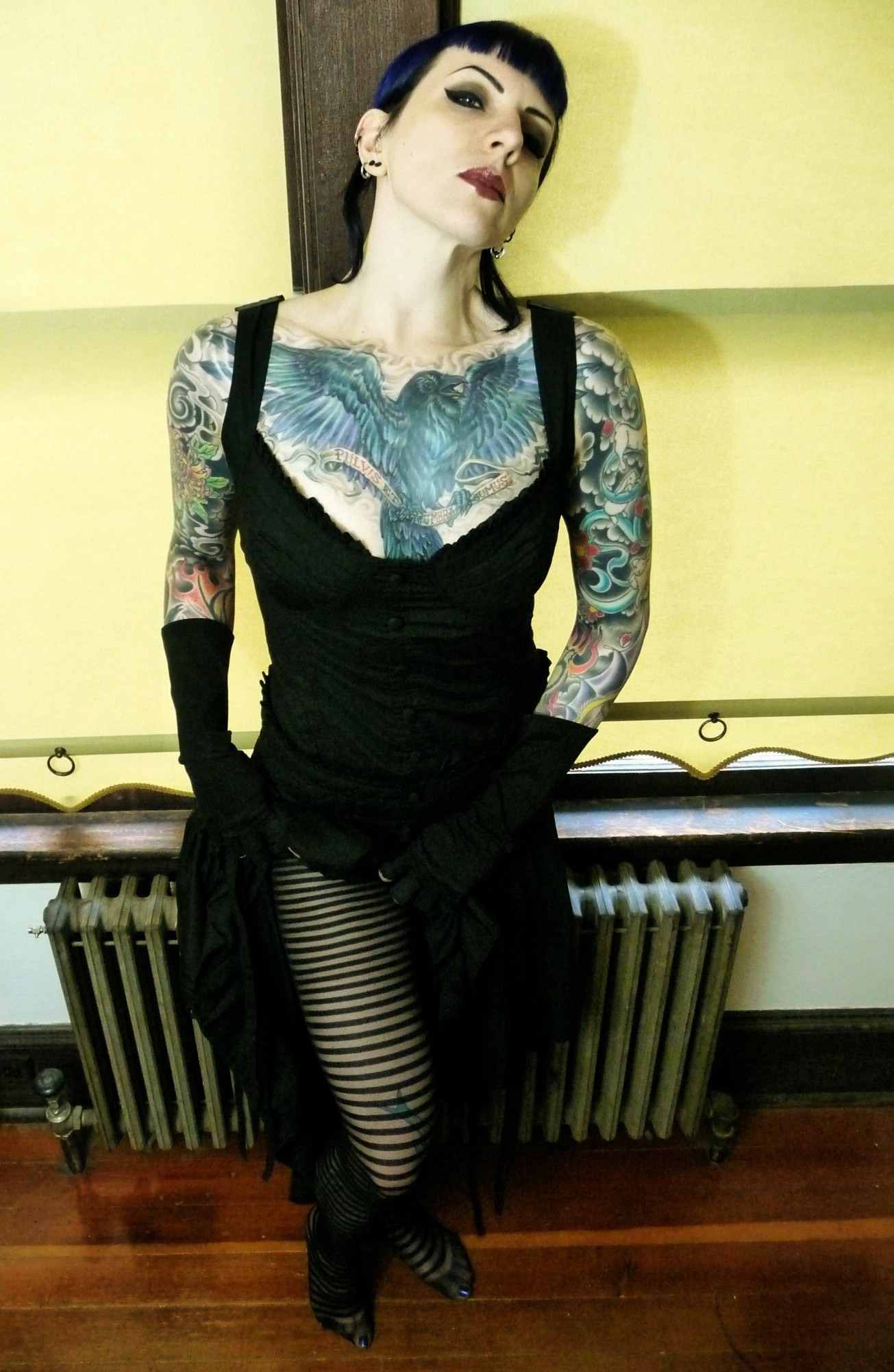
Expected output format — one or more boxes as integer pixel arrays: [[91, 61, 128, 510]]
[[163, 24, 650, 1348]]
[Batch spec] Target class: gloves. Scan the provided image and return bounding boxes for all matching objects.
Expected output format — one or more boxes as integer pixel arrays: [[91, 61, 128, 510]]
[[162, 618, 266, 828], [380, 713, 599, 918]]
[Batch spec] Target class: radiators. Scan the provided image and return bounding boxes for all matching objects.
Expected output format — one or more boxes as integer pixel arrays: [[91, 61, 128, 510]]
[[37, 860, 749, 1170]]
